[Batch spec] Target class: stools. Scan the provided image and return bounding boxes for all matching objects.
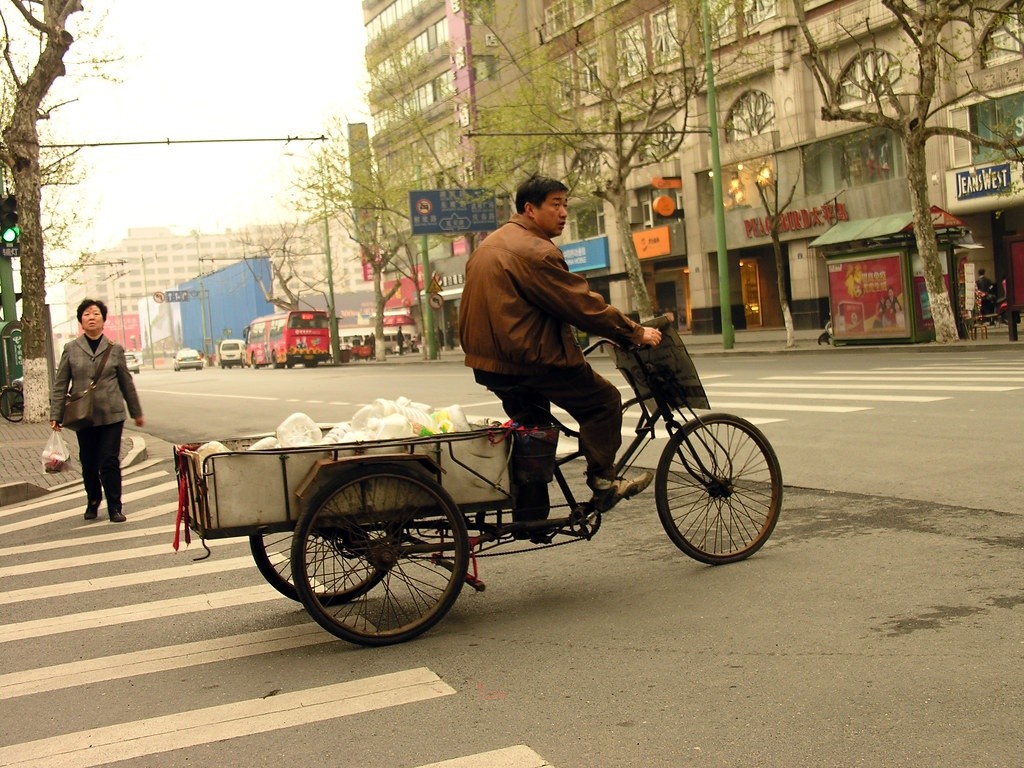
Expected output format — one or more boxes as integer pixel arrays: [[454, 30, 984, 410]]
[[970, 324, 987, 340]]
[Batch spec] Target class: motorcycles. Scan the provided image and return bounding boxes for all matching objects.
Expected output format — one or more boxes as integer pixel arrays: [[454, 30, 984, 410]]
[[350, 340, 371, 361]]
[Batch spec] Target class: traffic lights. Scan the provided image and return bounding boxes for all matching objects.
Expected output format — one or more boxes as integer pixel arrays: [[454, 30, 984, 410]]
[[1, 196, 19, 249]]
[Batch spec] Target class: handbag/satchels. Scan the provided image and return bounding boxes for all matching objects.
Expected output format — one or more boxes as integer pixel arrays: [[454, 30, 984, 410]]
[[61, 388, 96, 431], [42, 430, 72, 475]]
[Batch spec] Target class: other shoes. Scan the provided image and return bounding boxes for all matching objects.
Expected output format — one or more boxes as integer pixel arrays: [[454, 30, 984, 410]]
[[84, 498, 102, 520], [108, 506, 127, 522]]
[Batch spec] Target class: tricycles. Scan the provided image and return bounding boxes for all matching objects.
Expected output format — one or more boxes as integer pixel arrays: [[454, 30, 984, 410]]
[[172, 313, 784, 647]]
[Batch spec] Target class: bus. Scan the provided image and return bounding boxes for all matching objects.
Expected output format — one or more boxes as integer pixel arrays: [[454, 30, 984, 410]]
[[242, 310, 332, 369]]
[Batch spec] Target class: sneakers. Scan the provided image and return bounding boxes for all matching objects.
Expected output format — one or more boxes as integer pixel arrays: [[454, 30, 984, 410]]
[[512, 525, 556, 539], [588, 471, 653, 512]]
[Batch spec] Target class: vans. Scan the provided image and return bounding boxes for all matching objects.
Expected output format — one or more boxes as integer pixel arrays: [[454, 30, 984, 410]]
[[217, 339, 253, 369], [383, 333, 411, 355], [337, 335, 364, 350]]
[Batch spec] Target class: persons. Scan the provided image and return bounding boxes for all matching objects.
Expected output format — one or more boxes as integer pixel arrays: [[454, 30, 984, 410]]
[[349, 320, 455, 361], [975, 269, 993, 326], [872, 286, 906, 328], [457, 174, 662, 537], [49, 299, 144, 522]]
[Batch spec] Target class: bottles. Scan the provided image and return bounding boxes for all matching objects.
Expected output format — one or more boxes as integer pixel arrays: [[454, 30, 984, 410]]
[[196, 396, 471, 477]]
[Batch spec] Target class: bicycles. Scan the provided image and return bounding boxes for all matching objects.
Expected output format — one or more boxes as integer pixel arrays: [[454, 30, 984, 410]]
[[0, 375, 27, 423]]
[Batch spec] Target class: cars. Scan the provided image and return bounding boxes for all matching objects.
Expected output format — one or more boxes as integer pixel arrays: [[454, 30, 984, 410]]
[[123, 353, 140, 374], [172, 348, 204, 371]]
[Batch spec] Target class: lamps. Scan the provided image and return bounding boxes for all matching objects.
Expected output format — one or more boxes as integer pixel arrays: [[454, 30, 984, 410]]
[[706, 161, 774, 209]]
